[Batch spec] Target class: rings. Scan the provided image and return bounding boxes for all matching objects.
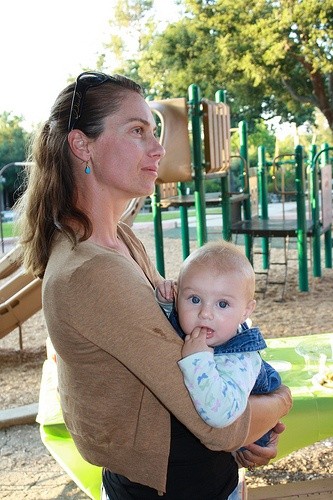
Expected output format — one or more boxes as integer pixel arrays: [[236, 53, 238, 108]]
[[247, 462, 258, 472]]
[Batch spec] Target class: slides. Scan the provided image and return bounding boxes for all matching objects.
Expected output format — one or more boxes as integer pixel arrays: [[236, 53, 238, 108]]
[[1, 96, 192, 341]]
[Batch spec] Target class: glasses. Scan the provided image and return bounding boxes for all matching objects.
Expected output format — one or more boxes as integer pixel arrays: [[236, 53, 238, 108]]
[[68, 70, 121, 131]]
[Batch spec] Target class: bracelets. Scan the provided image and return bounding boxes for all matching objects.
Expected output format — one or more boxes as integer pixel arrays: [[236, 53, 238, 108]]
[[271, 387, 292, 416]]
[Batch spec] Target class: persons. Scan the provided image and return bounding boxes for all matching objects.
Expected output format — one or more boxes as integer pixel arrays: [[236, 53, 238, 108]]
[[155, 241, 291, 470], [16, 73, 293, 500]]
